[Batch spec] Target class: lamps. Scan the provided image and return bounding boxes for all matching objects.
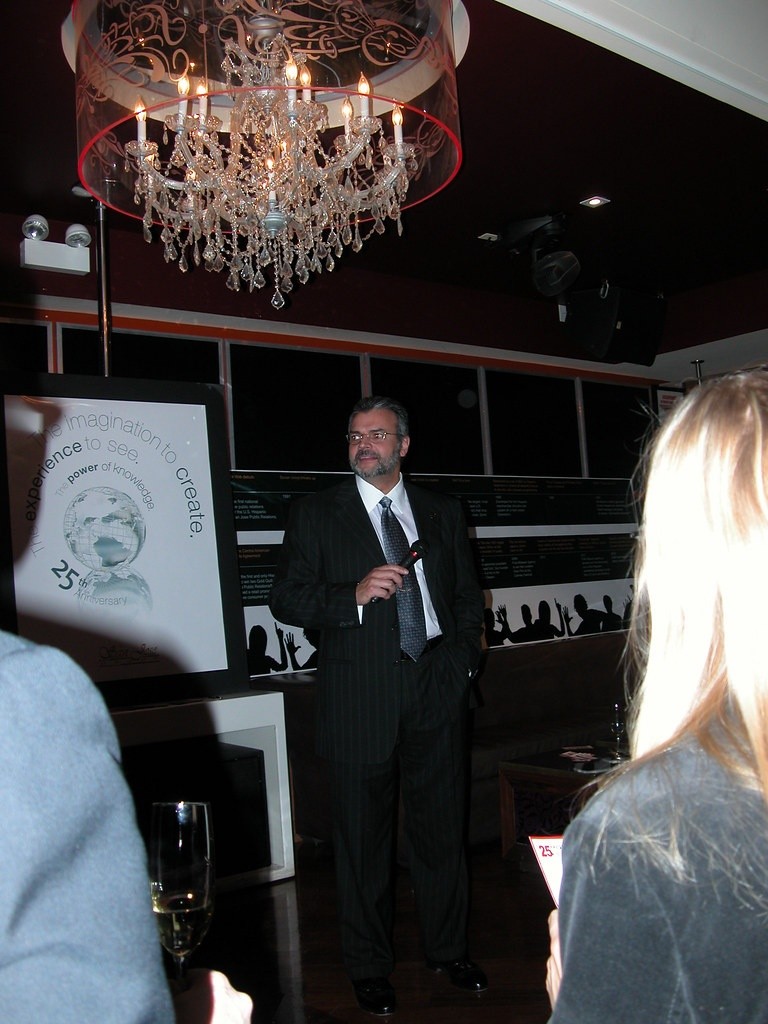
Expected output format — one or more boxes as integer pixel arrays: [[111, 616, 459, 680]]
[[19, 215, 91, 277], [61, 2, 471, 312]]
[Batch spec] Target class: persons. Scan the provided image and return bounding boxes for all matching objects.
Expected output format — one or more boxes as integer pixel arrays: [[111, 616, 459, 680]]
[[268, 393, 491, 1018], [548, 362, 767, 1024], [0, 629, 253, 1024]]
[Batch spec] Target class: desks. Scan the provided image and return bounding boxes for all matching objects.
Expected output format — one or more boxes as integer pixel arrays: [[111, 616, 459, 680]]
[[497, 738, 628, 882]]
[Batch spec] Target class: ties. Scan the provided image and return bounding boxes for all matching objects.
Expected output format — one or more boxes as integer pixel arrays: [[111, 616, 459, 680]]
[[378, 496, 427, 663]]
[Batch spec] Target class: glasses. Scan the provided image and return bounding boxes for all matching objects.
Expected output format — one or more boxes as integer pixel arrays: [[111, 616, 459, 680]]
[[345, 431, 404, 445]]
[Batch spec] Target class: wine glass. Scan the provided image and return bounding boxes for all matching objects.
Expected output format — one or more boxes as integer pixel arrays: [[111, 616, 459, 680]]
[[147, 798, 212, 994], [607, 703, 627, 763]]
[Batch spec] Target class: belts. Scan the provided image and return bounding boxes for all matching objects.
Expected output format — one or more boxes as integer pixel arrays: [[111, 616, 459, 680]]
[[401, 634, 447, 660]]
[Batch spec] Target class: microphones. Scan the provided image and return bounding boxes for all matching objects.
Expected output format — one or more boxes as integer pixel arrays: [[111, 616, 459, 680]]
[[372, 541, 431, 602]]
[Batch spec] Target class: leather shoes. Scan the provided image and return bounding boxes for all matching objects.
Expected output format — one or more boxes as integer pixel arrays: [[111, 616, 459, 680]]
[[425, 958, 489, 992], [351, 973, 398, 1016]]
